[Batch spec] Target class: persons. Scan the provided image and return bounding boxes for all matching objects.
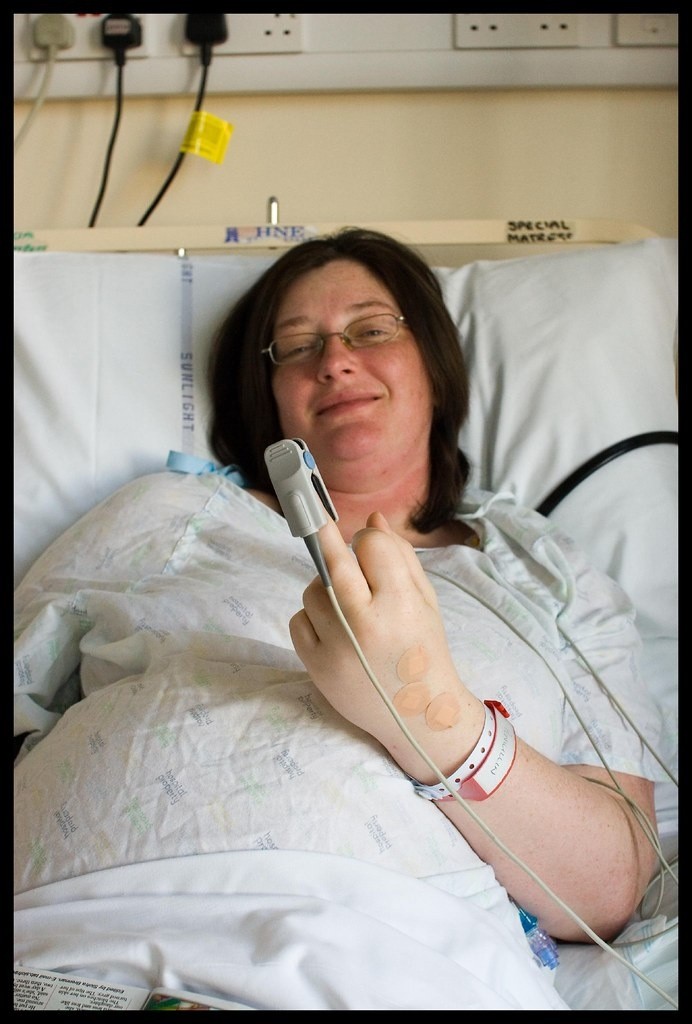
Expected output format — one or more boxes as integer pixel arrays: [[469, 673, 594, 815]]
[[14, 228, 662, 1010]]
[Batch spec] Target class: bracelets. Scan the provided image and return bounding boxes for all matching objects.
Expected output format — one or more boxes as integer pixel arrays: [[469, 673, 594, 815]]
[[411, 699, 518, 802]]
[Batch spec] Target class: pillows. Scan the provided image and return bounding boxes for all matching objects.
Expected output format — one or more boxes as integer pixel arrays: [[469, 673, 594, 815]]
[[15, 247, 680, 642]]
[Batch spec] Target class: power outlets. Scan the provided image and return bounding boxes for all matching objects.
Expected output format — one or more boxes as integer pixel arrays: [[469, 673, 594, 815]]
[[27, 13, 150, 61], [173, 12, 309, 58], [453, 12, 613, 49]]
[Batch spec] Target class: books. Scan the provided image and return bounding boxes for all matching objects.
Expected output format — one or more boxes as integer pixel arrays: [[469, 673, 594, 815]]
[[15, 963, 259, 1011]]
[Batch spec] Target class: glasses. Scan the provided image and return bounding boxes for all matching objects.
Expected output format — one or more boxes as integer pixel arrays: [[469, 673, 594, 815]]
[[262, 313, 406, 365]]
